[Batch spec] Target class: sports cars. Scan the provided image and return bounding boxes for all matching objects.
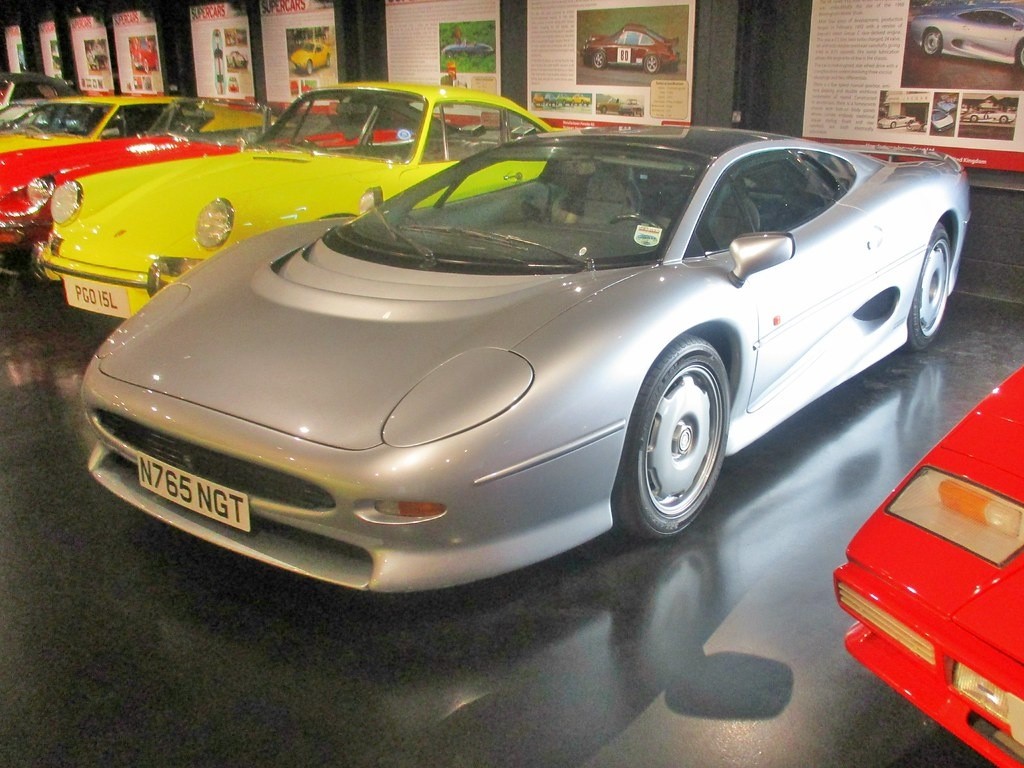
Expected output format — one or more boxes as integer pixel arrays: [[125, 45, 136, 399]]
[[76, 121, 970, 592]]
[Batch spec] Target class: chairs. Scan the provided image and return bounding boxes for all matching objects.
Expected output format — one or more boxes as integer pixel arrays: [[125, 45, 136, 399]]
[[708, 180, 762, 252], [577, 162, 643, 224]]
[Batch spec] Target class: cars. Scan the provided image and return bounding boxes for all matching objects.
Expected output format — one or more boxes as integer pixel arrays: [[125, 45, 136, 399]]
[[878, 114, 927, 132], [830, 361, 1024, 768], [596, 96, 645, 117], [911, 5, 1024, 72], [441, 41, 495, 57], [289, 40, 331, 74], [931, 93, 957, 133], [580, 22, 681, 73], [531, 91, 591, 110], [962, 109, 1016, 124], [0, 70, 563, 319]]
